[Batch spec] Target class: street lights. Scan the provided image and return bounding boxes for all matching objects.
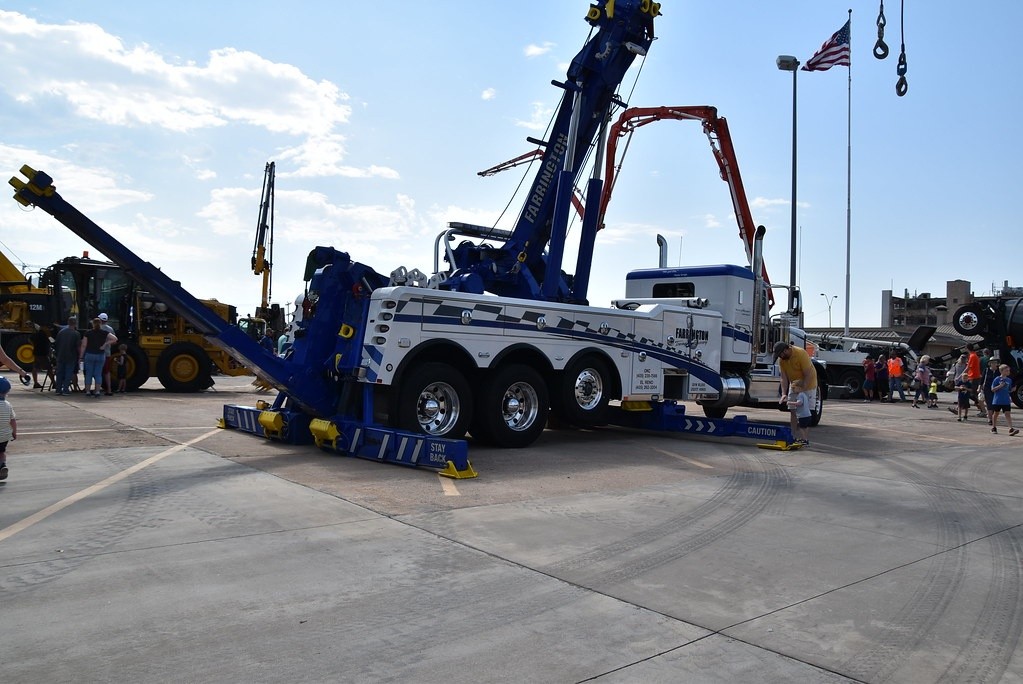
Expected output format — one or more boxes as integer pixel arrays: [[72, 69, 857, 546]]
[[820, 293, 837, 328], [775, 54, 801, 310]]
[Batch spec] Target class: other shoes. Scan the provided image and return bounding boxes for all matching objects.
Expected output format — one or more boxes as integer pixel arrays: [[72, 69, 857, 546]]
[[988, 420, 992, 425], [33, 382, 43, 388], [0, 464, 9, 480], [931, 404, 938, 408], [964, 416, 967, 420], [957, 418, 961, 421], [105, 392, 113, 396], [1009, 428, 1019, 436], [115, 390, 126, 394], [798, 439, 810, 447], [990, 427, 997, 434], [86, 391, 91, 397], [56, 389, 71, 396], [95, 392, 101, 398], [912, 403, 920, 409], [947, 406, 958, 415], [862, 399, 871, 403], [977, 410, 988, 417]]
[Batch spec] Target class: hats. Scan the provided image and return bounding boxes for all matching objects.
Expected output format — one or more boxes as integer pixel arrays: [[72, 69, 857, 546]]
[[865, 355, 872, 359], [0, 377, 11, 392], [774, 340, 787, 354], [986, 356, 1001, 365], [98, 313, 108, 322], [266, 328, 274, 333]]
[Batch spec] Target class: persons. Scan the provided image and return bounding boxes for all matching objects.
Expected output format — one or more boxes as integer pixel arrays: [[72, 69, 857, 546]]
[[0, 344, 27, 385], [278, 327, 290, 354], [787, 380, 812, 447], [260, 328, 276, 356], [946, 342, 1019, 436], [32, 313, 128, 398], [0, 375, 17, 480], [861, 342, 939, 408], [771, 341, 817, 444]]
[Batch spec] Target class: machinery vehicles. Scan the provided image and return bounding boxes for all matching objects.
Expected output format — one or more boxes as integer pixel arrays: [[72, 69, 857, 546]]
[[281, 0, 829, 448], [237, 161, 291, 356], [953, 296, 1023, 410], [0, 247, 268, 396]]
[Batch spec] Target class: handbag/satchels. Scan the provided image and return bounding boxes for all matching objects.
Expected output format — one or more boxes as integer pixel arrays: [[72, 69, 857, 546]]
[[910, 377, 921, 389], [978, 391, 985, 401], [945, 380, 955, 389]]
